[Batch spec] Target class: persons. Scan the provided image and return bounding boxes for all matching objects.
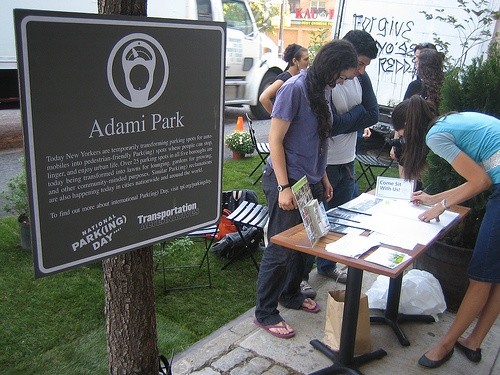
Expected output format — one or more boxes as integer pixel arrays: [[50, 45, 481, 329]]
[[391, 94, 500, 368], [258, 43, 310, 116], [300, 30, 380, 300], [389, 43, 446, 191], [254, 39, 358, 339]]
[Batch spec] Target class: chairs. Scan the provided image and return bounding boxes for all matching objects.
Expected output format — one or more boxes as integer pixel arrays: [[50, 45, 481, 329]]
[[155, 219, 221, 292], [221, 201, 272, 272], [355, 138, 400, 194], [244, 113, 270, 185]]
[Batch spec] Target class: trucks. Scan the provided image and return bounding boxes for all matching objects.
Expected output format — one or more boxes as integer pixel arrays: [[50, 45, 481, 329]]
[[0, 0, 290, 121], [331, 0, 500, 138]]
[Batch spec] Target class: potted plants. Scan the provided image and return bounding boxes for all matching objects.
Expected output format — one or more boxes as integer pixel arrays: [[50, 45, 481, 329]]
[[3, 156, 32, 249], [410, 44, 500, 299]]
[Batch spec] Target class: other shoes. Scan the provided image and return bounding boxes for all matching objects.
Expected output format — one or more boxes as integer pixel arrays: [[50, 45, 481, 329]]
[[455, 340, 481, 362], [323, 268, 348, 283], [418, 346, 454, 367], [299, 280, 316, 297]]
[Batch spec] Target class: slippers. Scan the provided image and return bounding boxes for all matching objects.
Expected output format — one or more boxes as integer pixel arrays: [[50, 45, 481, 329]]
[[254, 318, 295, 338], [301, 299, 320, 312]]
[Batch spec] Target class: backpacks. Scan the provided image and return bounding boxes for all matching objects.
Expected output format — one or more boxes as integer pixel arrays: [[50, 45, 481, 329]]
[[227, 189, 258, 230]]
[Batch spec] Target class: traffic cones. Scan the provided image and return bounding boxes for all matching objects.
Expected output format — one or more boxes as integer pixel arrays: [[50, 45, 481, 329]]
[[233, 116, 245, 132]]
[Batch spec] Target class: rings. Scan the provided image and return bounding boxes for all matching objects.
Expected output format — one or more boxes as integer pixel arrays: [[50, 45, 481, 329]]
[[330, 192, 333, 194], [422, 216, 424, 219]]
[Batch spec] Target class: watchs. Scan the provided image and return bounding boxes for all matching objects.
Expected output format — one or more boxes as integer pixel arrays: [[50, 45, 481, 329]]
[[441, 199, 447, 210], [277, 183, 290, 192]]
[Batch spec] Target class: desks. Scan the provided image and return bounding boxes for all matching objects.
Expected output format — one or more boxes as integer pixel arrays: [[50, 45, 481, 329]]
[[270, 188, 471, 375]]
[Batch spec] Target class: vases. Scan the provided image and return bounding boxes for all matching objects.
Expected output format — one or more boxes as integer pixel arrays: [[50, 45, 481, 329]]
[[233, 148, 245, 160]]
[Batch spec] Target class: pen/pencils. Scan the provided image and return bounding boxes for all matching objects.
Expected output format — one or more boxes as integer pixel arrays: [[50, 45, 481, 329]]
[[409, 184, 432, 203]]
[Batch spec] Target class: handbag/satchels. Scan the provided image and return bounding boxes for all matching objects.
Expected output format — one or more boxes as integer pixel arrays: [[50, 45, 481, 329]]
[[212, 226, 258, 253]]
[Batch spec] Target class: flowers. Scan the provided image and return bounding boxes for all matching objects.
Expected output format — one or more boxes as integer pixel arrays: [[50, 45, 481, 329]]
[[225, 130, 256, 156]]
